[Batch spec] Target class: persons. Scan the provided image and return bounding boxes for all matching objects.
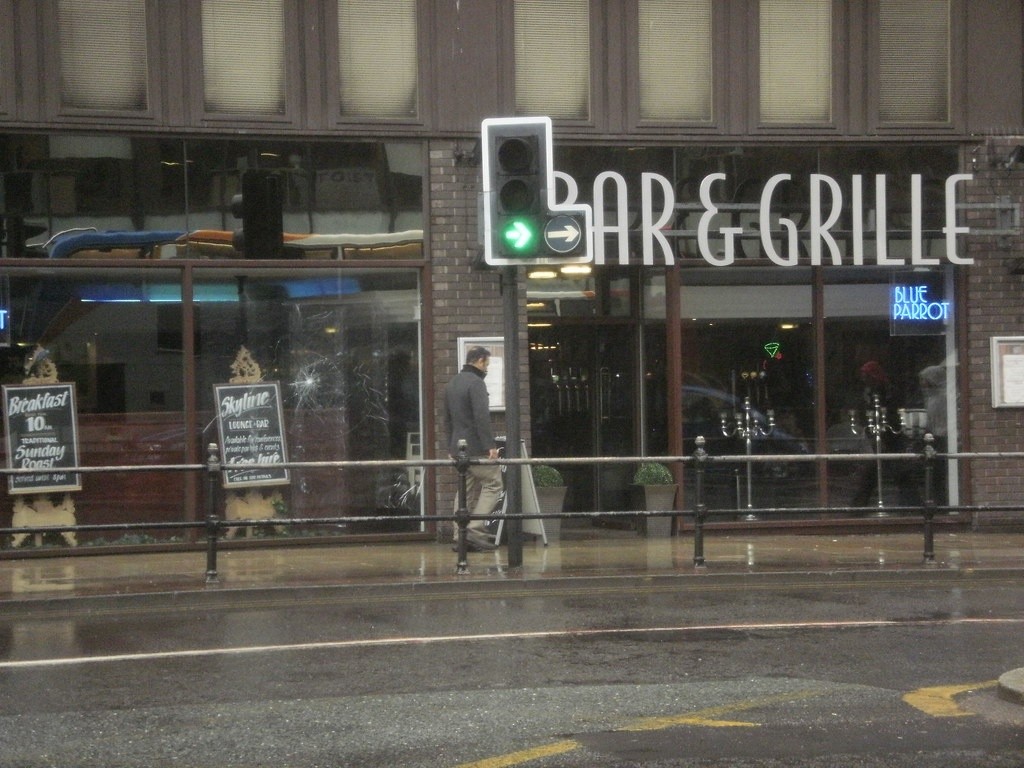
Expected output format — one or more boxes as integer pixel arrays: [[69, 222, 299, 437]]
[[920, 366, 947, 514], [849, 362, 925, 517], [443, 346, 503, 552]]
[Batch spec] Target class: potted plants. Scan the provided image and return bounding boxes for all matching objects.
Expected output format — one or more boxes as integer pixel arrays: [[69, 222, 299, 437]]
[[635, 464, 676, 539], [528, 464, 571, 528]]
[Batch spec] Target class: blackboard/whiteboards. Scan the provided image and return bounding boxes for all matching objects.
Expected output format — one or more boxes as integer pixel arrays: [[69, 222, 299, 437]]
[[215, 383, 287, 483], [4, 384, 79, 489]]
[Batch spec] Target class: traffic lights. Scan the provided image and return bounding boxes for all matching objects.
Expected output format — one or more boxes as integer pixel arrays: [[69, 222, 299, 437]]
[[230, 165, 286, 300], [478, 115, 596, 266]]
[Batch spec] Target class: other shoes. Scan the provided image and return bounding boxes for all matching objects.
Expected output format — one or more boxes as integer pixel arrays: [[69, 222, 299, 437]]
[[452, 541, 481, 552], [466, 530, 500, 549]]
